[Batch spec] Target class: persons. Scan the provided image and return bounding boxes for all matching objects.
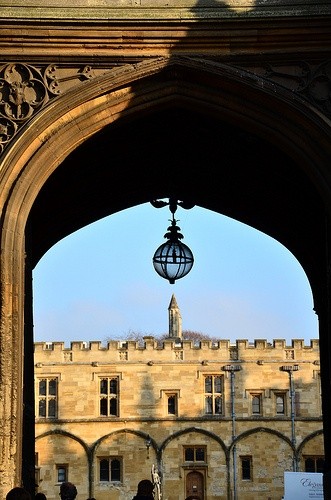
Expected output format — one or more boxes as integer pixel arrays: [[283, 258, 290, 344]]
[[6, 484, 47, 500], [59, 482, 78, 500], [132, 480, 154, 500]]
